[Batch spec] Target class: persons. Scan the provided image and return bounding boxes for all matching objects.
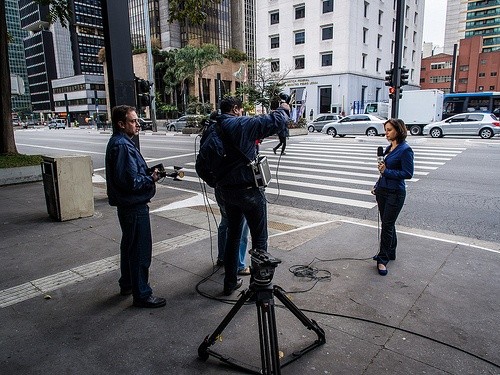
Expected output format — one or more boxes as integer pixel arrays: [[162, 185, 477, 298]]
[[105, 105, 166, 308], [371, 118, 414, 277], [195, 96, 291, 296]]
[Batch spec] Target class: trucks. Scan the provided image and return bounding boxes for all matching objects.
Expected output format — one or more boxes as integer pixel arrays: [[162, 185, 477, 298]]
[[363, 89, 444, 135]]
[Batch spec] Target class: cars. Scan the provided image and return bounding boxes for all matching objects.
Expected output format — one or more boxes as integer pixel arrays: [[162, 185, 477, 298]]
[[138, 118, 152, 131], [167, 115, 203, 131], [322, 114, 389, 138], [423, 113, 500, 139], [307, 115, 344, 132]]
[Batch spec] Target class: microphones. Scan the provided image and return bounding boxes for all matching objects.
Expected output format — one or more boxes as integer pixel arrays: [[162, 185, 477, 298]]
[[377, 147, 383, 165]]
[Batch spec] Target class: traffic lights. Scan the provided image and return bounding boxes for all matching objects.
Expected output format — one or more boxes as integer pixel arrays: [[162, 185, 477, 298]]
[[96, 98, 99, 102], [137, 78, 156, 109], [400, 68, 409, 86], [399, 89, 403, 99], [385, 68, 395, 86], [67, 113, 70, 117], [389, 88, 395, 98]]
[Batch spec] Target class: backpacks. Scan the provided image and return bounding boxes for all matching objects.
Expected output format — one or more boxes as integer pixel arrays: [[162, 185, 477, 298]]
[[195, 123, 225, 188]]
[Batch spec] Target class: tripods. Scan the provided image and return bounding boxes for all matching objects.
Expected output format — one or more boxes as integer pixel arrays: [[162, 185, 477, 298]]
[[197, 249, 325, 375]]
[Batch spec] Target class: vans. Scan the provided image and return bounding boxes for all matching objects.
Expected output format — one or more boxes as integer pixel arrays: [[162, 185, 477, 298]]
[[48, 119, 65, 129]]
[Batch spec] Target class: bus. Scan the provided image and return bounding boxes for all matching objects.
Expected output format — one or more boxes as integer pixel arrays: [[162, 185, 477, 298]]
[[442, 91, 500, 120]]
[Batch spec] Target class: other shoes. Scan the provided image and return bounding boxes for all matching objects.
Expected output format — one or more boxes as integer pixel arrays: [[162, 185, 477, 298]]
[[216, 259, 223, 266], [373, 255, 378, 260], [281, 153, 286, 155], [133, 296, 166, 308], [238, 267, 250, 275], [120, 287, 132, 295], [273, 148, 276, 154], [377, 262, 387, 276], [223, 278, 243, 296]]
